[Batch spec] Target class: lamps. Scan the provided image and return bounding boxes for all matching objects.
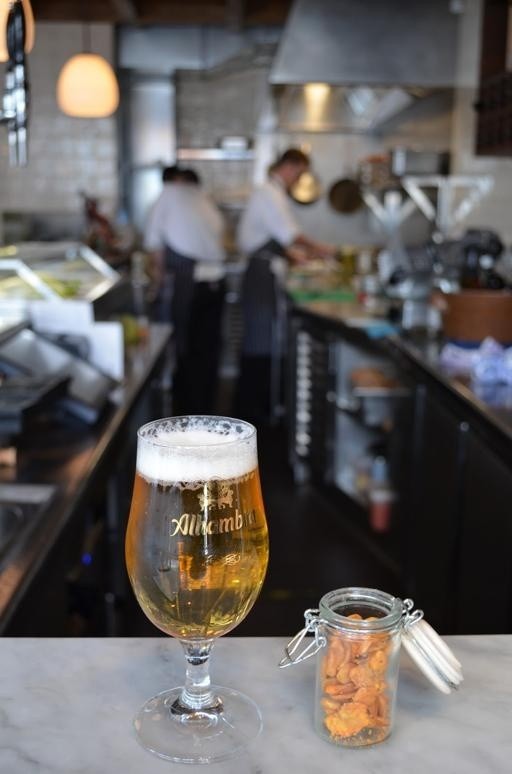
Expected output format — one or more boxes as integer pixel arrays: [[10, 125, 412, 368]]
[[57, 0, 121, 119]]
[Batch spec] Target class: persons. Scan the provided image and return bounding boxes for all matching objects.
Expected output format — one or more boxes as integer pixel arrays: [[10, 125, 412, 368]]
[[232, 145, 337, 432], [142, 164, 236, 416]]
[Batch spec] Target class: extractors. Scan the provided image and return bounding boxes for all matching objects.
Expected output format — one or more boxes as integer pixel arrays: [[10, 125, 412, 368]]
[[253, 0, 465, 140]]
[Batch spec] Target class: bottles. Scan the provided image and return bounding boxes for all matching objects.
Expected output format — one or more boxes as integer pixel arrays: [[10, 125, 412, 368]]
[[281, 587, 463, 748]]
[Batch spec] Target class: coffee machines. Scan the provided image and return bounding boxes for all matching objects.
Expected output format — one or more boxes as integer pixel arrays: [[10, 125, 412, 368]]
[[382, 226, 508, 338]]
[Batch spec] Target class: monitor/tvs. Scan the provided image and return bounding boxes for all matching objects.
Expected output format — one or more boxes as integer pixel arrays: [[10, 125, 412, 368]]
[[0, 320, 118, 425]]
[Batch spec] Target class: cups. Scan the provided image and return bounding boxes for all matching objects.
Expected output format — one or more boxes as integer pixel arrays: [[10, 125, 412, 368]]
[[366, 488, 395, 528]]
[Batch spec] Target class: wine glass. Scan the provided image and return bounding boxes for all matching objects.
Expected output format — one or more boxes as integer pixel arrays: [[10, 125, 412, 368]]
[[124, 410, 270, 764]]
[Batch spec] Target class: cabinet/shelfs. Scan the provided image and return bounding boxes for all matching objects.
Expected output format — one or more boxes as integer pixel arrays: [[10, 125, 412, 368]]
[[172, 59, 263, 275]]
